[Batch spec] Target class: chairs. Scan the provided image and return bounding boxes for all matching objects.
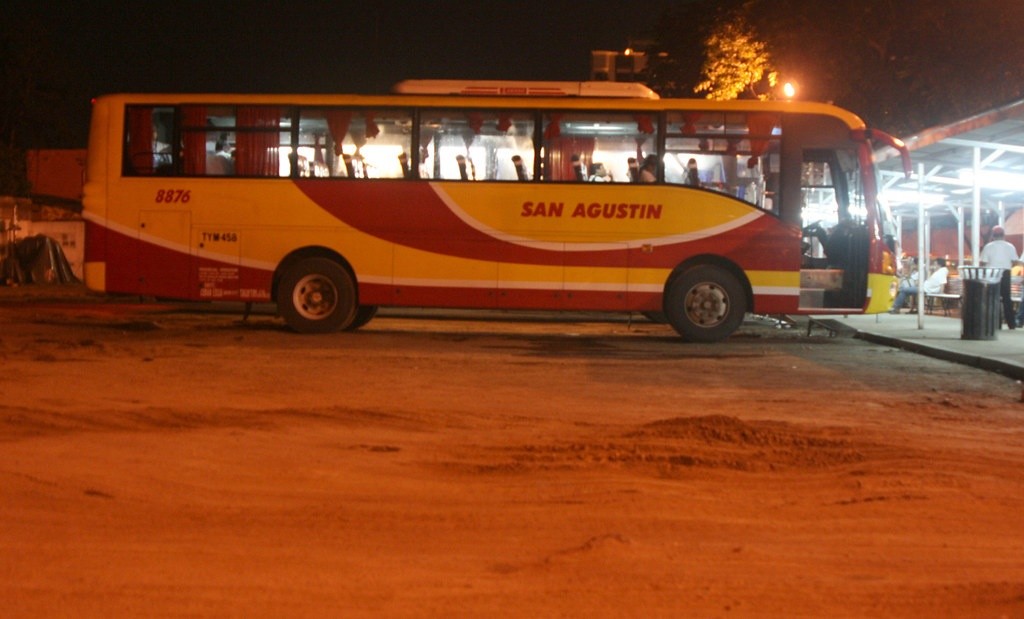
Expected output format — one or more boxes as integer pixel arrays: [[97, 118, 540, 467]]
[[288, 152, 701, 187]]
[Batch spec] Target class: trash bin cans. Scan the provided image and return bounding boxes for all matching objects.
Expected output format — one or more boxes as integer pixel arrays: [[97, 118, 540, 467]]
[[956, 263, 1006, 341]]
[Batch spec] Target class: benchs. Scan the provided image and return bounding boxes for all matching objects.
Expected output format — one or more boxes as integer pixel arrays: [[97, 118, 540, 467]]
[[1000, 277, 1024, 315], [906, 277, 961, 316]]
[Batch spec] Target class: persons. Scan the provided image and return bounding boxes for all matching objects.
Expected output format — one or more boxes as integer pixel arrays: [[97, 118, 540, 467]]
[[588, 154, 658, 183], [886, 225, 1024, 330]]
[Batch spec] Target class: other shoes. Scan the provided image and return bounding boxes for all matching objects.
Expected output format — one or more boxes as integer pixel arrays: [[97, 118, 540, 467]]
[[1008, 323, 1017, 329], [888, 309, 900, 314]]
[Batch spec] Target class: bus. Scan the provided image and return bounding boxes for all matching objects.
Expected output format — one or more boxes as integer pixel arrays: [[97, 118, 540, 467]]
[[80, 78, 913, 343]]
